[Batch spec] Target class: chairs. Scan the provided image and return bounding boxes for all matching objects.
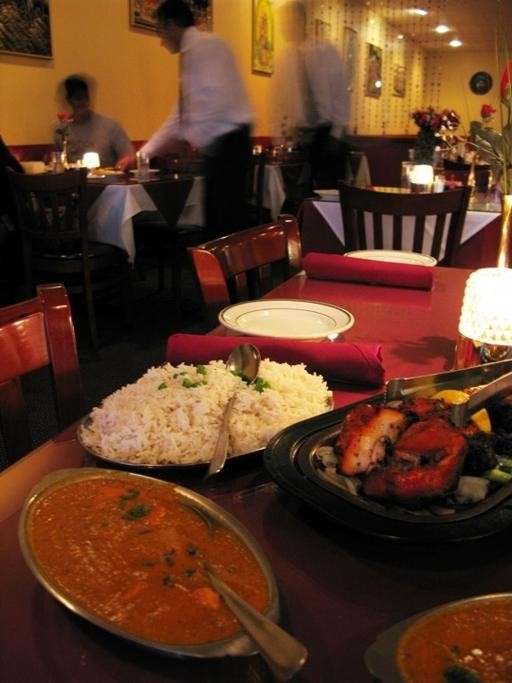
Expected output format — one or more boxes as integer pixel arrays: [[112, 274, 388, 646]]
[[1, 161, 98, 355], [247, 154, 271, 227], [188, 214, 303, 334], [337, 181, 473, 268], [0, 281, 81, 473]]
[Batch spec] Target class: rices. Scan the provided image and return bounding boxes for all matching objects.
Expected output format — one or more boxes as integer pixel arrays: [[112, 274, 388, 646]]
[[79, 358, 334, 466]]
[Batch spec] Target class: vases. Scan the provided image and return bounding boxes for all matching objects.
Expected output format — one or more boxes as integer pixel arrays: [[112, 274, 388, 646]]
[[60, 138, 73, 170]]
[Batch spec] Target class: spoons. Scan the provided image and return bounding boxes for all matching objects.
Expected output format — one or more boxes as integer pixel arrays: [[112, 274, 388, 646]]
[[204, 342, 261, 475]]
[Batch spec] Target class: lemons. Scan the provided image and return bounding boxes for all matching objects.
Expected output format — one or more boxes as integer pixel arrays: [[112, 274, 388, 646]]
[[430, 389, 493, 434]]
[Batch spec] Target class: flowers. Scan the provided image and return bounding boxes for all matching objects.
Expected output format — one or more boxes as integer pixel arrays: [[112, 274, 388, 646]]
[[56, 112, 77, 138], [405, 1, 512, 192]]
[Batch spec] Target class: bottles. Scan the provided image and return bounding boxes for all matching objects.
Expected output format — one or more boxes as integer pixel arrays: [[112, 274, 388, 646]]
[[272, 146, 283, 164], [138, 151, 149, 178]]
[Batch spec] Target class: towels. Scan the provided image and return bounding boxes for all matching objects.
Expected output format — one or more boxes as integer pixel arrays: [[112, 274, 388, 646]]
[[165, 332, 385, 392], [304, 252, 433, 290]]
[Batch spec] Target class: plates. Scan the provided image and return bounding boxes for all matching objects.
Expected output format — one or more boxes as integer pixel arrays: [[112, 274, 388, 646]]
[[22, 467, 283, 661], [76, 390, 333, 472], [363, 592, 511, 682], [343, 250, 438, 268], [219, 299, 354, 339], [262, 393, 511, 539]]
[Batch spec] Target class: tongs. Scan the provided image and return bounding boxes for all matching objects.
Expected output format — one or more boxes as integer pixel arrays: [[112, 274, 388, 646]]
[[383, 359, 511, 427]]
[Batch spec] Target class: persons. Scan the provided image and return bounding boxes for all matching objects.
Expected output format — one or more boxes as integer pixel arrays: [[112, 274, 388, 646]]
[[113, 0, 252, 236], [269, 2, 352, 226], [52, 72, 137, 173]]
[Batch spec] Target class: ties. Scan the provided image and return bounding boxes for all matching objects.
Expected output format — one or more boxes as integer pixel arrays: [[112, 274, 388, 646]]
[[296, 52, 323, 129], [177, 52, 187, 125]]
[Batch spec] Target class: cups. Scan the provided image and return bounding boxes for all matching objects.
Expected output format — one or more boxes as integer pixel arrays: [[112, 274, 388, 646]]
[[51, 150, 66, 175]]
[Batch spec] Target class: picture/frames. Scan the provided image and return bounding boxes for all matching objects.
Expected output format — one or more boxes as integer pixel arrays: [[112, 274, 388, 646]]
[[314, 20, 406, 100], [0, 0, 54, 60], [128, 0, 214, 39]]
